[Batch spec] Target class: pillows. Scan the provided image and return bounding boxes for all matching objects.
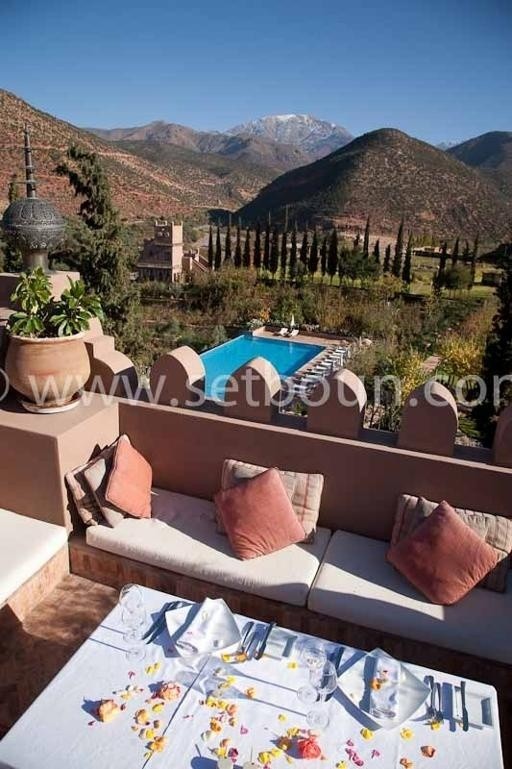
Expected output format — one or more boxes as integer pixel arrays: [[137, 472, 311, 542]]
[[385, 491, 512, 606], [212, 458, 325, 562], [64, 432, 154, 531]]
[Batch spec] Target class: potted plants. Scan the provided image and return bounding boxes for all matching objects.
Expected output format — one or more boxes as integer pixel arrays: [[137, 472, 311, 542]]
[[4, 260, 107, 407]]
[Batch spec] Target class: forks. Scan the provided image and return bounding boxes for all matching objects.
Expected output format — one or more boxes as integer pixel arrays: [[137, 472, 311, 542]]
[[436, 683, 443, 722]]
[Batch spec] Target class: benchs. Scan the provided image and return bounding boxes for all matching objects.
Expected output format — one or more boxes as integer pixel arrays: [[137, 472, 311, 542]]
[[66, 487, 511, 701]]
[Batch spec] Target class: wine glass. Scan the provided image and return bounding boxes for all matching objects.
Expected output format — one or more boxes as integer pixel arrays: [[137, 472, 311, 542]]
[[296, 638, 338, 729], [118, 583, 146, 663]]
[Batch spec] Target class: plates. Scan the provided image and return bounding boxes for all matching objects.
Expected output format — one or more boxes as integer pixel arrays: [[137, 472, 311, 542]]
[[165, 598, 242, 659], [451, 684, 495, 729], [337, 647, 432, 730], [256, 627, 298, 661]]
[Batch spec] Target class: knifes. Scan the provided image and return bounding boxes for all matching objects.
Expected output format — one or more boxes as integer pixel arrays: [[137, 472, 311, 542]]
[[255, 622, 277, 660], [146, 601, 180, 644], [325, 647, 346, 702], [461, 681, 468, 732]]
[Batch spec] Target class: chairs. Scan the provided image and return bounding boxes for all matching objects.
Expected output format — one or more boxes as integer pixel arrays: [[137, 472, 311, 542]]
[[274, 327, 299, 338], [284, 344, 350, 396]]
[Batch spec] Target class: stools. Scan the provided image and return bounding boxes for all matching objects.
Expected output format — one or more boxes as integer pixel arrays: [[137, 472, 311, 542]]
[[0, 507, 69, 636]]
